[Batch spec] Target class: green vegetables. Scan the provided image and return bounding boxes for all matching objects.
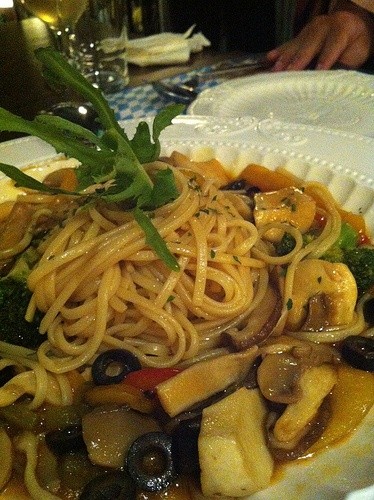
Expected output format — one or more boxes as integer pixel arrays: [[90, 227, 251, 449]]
[[0, 46, 188, 271]]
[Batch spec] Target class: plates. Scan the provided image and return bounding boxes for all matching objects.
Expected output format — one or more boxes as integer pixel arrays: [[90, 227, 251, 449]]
[[186, 67, 374, 139], [0, 111, 374, 500]]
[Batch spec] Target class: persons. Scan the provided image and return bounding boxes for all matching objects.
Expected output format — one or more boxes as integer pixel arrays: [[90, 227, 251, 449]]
[[264, 0, 374, 75]]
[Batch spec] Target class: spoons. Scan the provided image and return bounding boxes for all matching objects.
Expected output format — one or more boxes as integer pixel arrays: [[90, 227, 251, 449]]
[[149, 59, 278, 105]]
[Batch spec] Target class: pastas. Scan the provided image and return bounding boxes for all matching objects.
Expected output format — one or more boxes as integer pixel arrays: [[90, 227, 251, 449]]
[[2, 152, 373, 500]]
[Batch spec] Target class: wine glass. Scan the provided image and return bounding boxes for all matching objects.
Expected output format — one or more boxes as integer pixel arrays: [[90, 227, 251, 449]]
[[14, 0, 92, 71]]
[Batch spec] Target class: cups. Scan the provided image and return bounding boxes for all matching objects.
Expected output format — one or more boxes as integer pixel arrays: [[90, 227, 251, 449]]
[[66, 1, 129, 93]]
